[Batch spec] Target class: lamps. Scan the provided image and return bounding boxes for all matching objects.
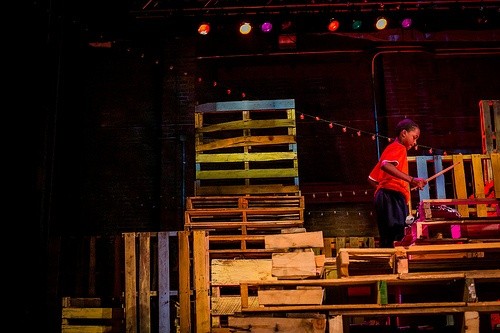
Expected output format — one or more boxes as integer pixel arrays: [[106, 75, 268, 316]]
[[198, 14, 412, 34]]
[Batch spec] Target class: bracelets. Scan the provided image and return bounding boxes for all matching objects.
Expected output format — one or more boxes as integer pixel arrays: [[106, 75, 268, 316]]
[[409, 176, 415, 183]]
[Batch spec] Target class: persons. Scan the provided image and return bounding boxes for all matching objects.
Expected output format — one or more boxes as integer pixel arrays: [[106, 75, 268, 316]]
[[368, 122, 428, 248]]
